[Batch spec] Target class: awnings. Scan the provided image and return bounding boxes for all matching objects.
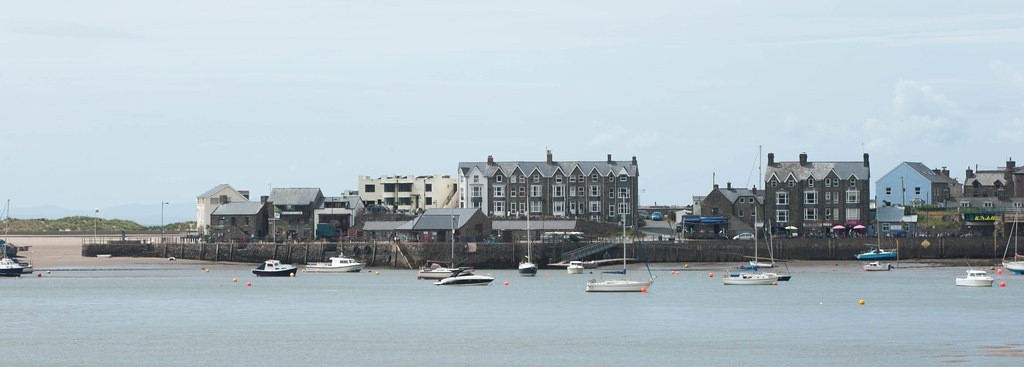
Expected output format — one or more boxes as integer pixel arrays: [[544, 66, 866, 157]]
[[844, 220, 858, 225]]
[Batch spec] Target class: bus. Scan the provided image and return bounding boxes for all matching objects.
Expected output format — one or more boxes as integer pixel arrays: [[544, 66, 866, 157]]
[[545, 231, 583, 241]]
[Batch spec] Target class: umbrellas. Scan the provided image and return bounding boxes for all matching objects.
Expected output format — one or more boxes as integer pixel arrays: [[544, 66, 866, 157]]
[[833, 224, 845, 238], [785, 226, 798, 236], [853, 224, 866, 238]]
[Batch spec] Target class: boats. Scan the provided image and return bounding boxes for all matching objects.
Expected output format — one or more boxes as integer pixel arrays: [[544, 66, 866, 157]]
[[304, 250, 367, 275], [251, 259, 299, 277], [434, 266, 495, 288], [568, 260, 585, 274], [97, 254, 113, 259], [863, 262, 895, 272], [954, 261, 994, 286]]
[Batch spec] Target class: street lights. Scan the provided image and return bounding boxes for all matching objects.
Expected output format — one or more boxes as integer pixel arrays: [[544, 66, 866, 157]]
[[900, 177, 905, 205], [94, 209, 99, 242], [331, 197, 337, 245], [161, 201, 169, 240]]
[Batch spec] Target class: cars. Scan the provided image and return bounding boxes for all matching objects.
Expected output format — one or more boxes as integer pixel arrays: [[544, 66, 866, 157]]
[[731, 232, 756, 241], [885, 229, 908, 237], [652, 212, 662, 221], [917, 230, 983, 238]]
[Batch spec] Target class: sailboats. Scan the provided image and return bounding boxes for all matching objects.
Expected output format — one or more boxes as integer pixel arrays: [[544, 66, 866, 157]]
[[584, 212, 656, 292], [854, 194, 897, 262], [0, 199, 26, 278], [416, 215, 476, 280], [518, 194, 540, 276], [1001, 203, 1024, 275], [720, 206, 792, 286]]
